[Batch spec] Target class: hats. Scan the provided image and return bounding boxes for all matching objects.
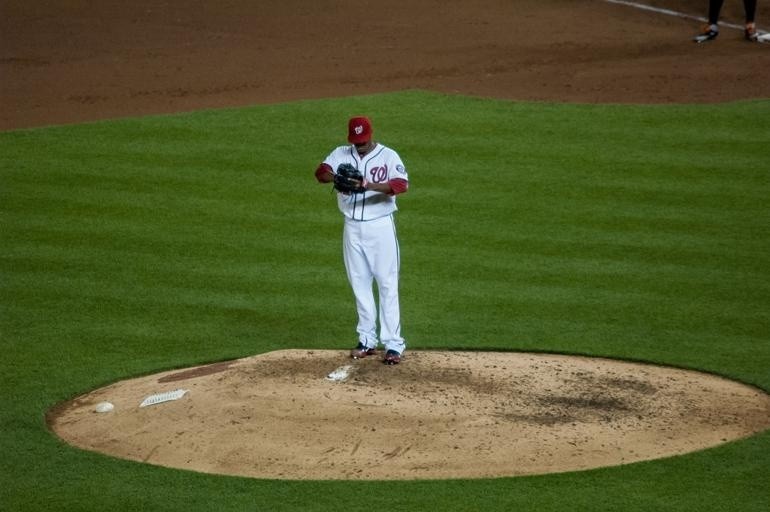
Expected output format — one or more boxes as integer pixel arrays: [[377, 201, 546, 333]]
[[348, 117, 371, 144]]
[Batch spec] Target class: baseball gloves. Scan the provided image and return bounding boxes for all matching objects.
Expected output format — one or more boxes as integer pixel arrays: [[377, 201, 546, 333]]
[[333, 163, 368, 195]]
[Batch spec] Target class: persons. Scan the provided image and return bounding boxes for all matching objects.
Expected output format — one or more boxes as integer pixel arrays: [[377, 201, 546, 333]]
[[313, 116, 409, 364], [691, 0, 770, 45]]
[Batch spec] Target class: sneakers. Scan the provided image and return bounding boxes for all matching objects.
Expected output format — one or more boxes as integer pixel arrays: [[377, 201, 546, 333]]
[[351, 342, 375, 358], [384, 350, 401, 364]]
